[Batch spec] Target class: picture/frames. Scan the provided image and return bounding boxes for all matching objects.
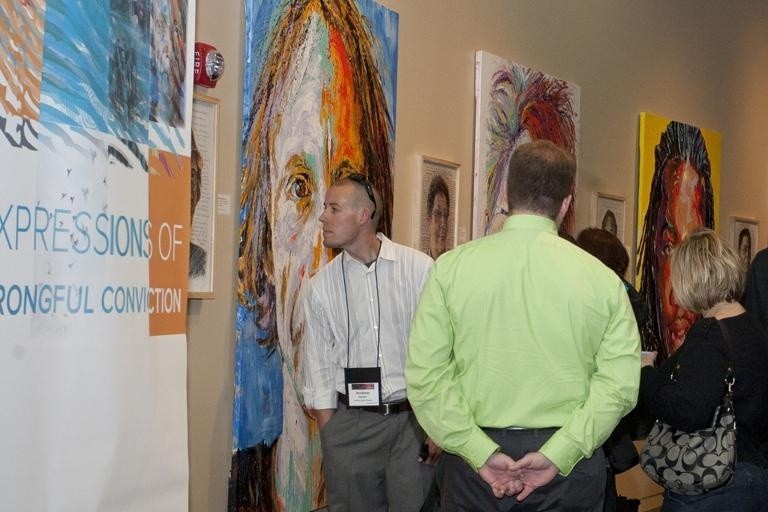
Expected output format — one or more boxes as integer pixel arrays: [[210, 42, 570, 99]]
[[413, 154, 462, 263], [727, 214, 759, 273], [591, 192, 626, 248], [186, 90, 222, 300]]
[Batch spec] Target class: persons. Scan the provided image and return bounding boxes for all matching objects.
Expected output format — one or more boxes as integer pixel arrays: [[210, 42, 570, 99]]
[[737, 228, 753, 276], [188, 134, 209, 282], [738, 246, 768, 328], [421, 173, 453, 260], [232, 2, 393, 510], [294, 172, 443, 510], [637, 228, 768, 512], [574, 225, 651, 512], [599, 209, 619, 235], [403, 140, 644, 511], [637, 117, 717, 368]]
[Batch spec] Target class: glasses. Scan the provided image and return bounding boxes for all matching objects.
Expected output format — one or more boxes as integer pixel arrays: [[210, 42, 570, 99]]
[[346, 172, 377, 221]]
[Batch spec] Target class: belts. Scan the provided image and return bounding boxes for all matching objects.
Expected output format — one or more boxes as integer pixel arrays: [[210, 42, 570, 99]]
[[335, 389, 411, 416]]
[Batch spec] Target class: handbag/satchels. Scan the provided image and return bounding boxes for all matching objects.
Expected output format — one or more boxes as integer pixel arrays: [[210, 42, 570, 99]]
[[638, 316, 739, 495], [602, 432, 642, 475]]
